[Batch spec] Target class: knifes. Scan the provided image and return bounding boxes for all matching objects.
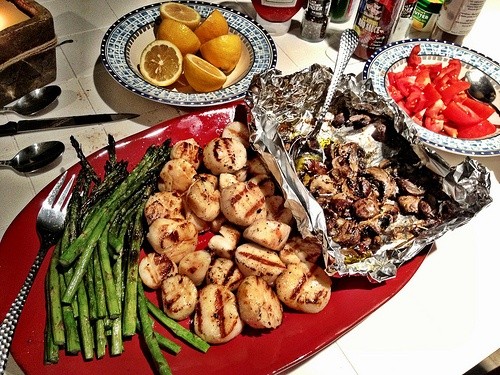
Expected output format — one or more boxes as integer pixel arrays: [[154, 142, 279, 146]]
[[0, 112, 141, 139]]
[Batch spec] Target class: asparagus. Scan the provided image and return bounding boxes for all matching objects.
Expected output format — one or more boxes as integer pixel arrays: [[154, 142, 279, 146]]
[[45, 133, 210, 375]]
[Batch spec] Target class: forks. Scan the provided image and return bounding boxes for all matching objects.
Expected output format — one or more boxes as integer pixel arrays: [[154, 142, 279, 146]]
[[0, 169, 96, 374]]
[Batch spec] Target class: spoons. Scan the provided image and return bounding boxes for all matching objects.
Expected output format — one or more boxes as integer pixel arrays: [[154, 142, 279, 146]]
[[463, 68, 500, 122], [0, 84, 61, 117], [0, 141, 66, 175], [288, 28, 361, 180]]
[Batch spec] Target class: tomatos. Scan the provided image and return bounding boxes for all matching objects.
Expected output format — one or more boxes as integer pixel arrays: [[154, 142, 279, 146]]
[[388, 46, 496, 138]]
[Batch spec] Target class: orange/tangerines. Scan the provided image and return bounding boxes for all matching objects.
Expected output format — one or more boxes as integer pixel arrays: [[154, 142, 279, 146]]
[[140, 3, 242, 92]]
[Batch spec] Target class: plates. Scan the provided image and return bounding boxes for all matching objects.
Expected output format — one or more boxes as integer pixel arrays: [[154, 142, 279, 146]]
[[1, 91, 459, 375], [99, 0, 277, 106], [363, 38, 500, 159]]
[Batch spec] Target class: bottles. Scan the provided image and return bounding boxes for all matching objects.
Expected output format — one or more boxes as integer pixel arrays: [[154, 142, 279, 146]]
[[400, 0, 418, 18], [429, 0, 489, 46], [253, 0, 307, 37], [408, 0, 446, 34], [347, 0, 405, 60], [300, 0, 332, 42], [328, 0, 359, 23]]
